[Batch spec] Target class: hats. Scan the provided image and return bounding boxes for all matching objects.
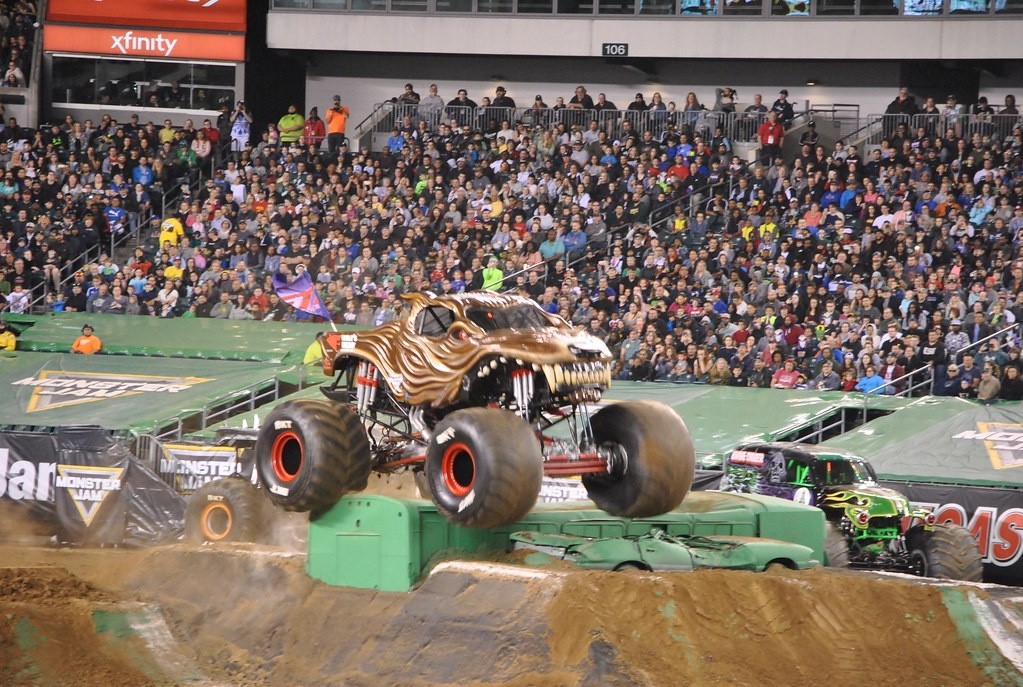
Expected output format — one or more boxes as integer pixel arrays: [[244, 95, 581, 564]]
[[5, 113, 1016, 382]]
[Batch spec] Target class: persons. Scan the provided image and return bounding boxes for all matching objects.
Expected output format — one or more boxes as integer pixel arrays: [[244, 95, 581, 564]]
[[0, 0, 1023, 405], [69, 323, 102, 354], [0, 320, 16, 351]]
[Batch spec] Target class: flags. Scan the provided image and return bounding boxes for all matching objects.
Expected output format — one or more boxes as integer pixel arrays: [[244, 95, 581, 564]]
[[272, 270, 332, 323]]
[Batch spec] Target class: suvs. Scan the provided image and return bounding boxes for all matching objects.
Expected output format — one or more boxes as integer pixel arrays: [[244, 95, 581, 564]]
[[183, 425, 283, 551], [255, 287, 696, 527], [725, 441, 984, 583]]
